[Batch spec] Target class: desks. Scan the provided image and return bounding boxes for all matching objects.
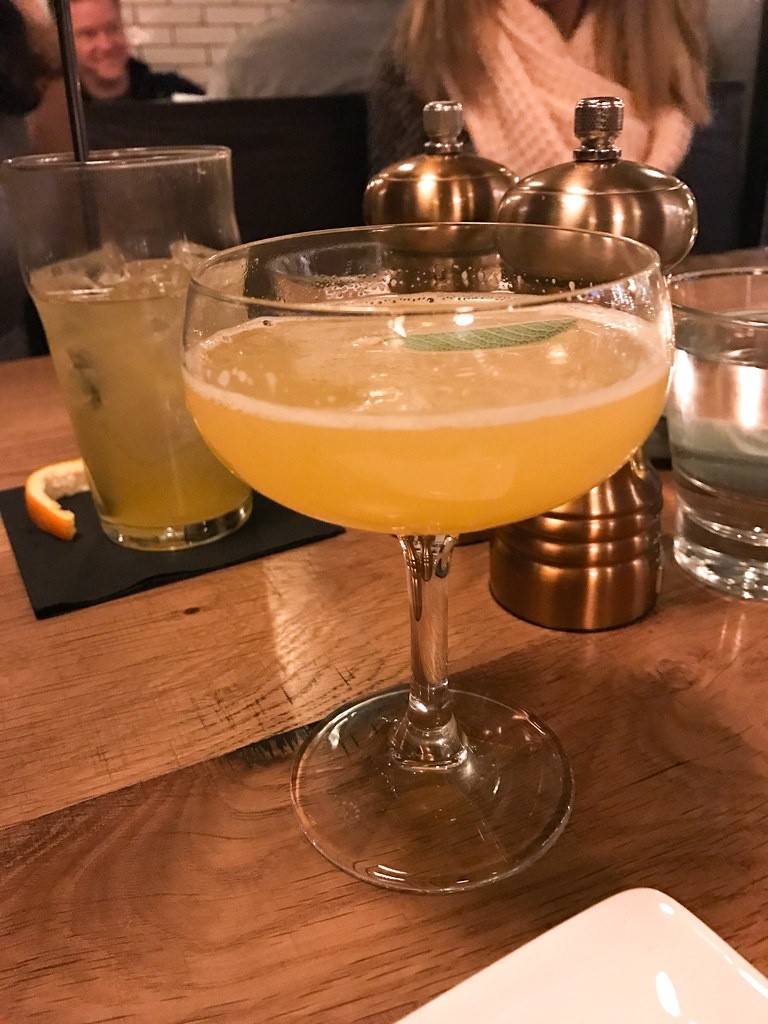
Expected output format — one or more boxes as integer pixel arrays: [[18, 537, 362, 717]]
[[0, 242, 768, 1024]]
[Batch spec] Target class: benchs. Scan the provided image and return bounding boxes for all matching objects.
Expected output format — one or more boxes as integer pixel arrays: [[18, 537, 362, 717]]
[[82, 93, 369, 243]]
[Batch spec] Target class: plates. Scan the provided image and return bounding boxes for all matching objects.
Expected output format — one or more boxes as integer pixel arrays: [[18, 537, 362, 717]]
[[396, 887, 768, 1024]]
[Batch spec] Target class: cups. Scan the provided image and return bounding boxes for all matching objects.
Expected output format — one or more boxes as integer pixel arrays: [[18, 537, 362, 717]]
[[664, 267, 768, 602], [0, 144, 254, 552]]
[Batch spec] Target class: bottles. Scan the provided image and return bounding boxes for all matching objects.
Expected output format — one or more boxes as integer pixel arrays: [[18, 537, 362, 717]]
[[364, 97, 526, 296], [487, 92, 702, 632]]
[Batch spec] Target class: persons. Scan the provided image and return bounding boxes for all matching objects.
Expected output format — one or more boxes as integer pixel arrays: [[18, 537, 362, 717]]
[[62, 0, 207, 105], [1, 0, 64, 361], [367, 0, 719, 257]]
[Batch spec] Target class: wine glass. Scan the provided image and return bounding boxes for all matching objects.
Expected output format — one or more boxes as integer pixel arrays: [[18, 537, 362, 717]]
[[176, 221, 678, 902]]
